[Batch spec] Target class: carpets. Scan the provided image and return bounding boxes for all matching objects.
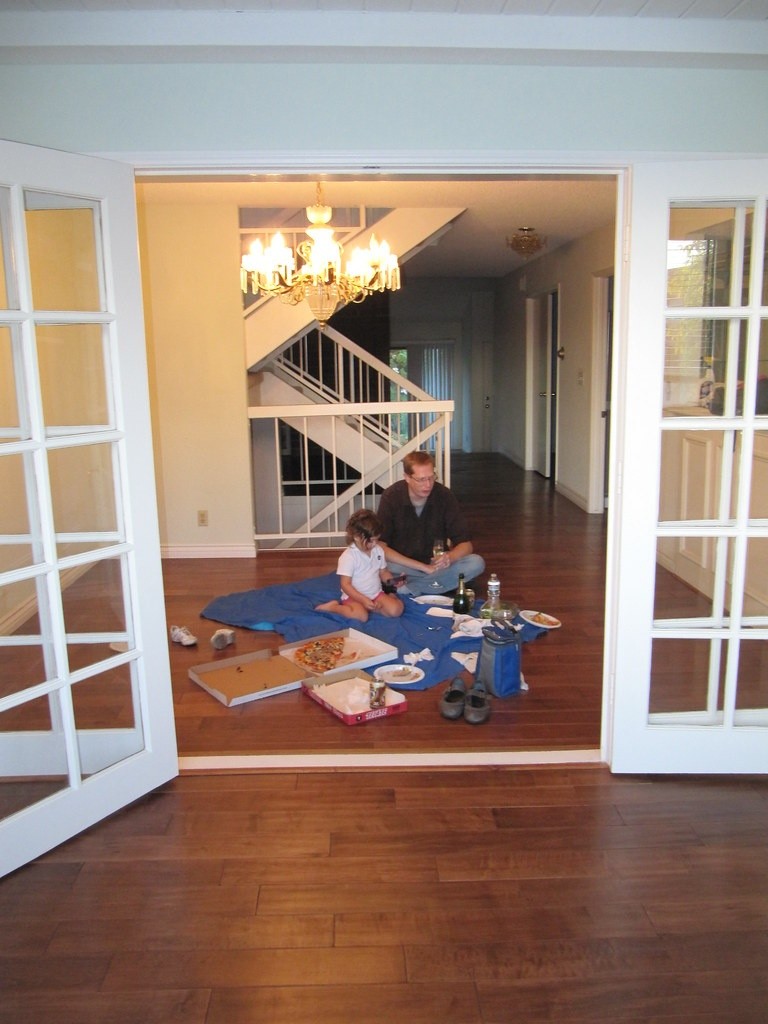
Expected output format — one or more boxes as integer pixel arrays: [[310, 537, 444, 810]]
[[199, 570, 549, 692]]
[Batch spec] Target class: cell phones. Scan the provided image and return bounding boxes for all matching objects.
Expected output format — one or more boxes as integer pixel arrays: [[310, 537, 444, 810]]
[[387, 576, 405, 586]]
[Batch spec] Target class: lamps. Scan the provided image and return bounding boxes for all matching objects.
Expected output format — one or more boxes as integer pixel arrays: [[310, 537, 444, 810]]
[[240, 181, 400, 334], [505, 225, 549, 262]]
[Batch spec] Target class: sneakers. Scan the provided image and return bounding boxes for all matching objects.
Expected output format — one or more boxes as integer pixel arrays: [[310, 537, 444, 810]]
[[208, 628, 237, 649], [169, 624, 200, 648]]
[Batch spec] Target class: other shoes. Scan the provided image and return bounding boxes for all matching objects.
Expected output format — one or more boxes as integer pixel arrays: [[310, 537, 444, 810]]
[[463, 679, 492, 726], [439, 677, 466, 721]]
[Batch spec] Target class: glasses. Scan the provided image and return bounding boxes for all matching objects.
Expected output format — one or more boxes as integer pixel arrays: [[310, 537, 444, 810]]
[[407, 472, 440, 486]]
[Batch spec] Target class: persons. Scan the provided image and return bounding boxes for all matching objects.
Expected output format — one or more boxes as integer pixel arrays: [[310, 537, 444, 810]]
[[375, 450, 485, 596], [315, 508, 406, 623]]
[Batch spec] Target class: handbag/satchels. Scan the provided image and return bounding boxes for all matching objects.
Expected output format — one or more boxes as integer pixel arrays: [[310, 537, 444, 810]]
[[475, 618, 523, 700]]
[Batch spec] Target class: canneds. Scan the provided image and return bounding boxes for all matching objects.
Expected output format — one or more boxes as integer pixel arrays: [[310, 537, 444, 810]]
[[463, 588, 475, 611], [369, 676, 388, 710]]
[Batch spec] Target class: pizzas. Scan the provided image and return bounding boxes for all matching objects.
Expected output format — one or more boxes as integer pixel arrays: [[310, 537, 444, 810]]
[[294, 636, 359, 673], [531, 613, 559, 627]]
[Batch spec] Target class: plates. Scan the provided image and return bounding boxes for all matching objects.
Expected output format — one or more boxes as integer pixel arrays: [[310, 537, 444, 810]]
[[373, 663, 427, 684], [519, 610, 562, 631]]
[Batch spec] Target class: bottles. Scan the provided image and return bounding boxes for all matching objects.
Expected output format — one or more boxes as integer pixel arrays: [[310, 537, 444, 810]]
[[452, 573, 470, 624], [487, 573, 500, 609]]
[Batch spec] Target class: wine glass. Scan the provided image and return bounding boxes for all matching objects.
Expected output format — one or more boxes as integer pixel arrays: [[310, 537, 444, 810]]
[[427, 539, 446, 589]]
